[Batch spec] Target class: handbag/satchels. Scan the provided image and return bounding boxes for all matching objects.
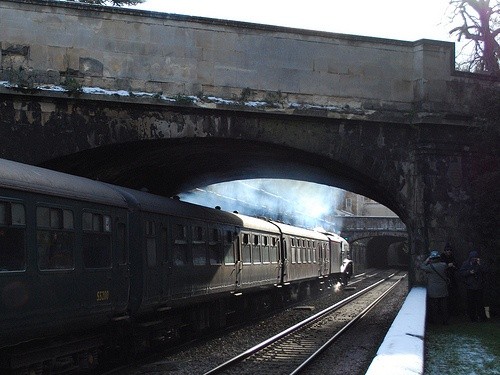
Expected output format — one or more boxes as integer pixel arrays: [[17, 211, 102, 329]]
[[458, 270, 476, 295]]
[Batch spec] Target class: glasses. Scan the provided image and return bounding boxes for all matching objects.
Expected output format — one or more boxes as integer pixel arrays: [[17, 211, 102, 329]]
[[472, 259, 476, 260]]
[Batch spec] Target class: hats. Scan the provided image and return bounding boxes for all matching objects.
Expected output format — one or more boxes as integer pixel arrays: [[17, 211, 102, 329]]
[[444, 246, 452, 251], [429, 251, 440, 258], [469, 251, 480, 257]]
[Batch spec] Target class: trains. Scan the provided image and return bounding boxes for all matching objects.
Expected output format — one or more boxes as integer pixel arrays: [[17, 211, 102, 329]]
[[1, 156, 355, 350]]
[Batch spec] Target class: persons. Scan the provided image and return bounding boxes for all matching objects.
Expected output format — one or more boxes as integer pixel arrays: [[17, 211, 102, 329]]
[[456, 251, 487, 322], [440, 251, 457, 294], [421, 252, 449, 326]]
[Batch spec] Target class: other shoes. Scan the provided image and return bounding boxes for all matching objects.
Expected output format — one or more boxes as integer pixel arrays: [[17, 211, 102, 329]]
[[429, 321, 447, 325], [472, 317, 487, 322]]
